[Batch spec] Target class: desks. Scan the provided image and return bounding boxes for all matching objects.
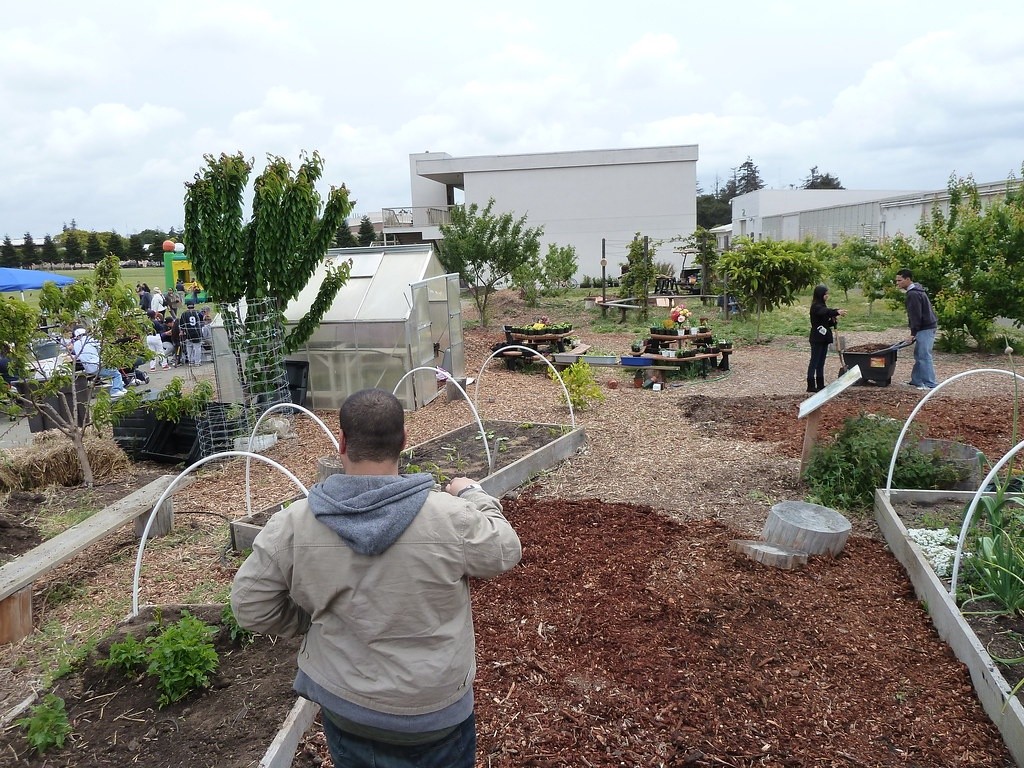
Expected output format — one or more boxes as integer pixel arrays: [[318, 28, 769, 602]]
[[33, 312, 147, 330], [650, 331, 711, 349], [510, 330, 575, 361]]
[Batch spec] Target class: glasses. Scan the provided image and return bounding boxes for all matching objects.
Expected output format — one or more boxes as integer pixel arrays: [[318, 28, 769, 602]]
[[201, 311, 205, 312], [188, 307, 194, 309]]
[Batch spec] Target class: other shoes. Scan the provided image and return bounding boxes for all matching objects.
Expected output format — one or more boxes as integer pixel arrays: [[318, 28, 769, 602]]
[[907, 382, 923, 391], [189, 362, 202, 367], [924, 386, 935, 391]]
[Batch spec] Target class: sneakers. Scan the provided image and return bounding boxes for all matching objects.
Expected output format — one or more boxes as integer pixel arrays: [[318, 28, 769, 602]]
[[111, 388, 127, 396], [151, 367, 156, 372], [163, 365, 171, 371], [142, 371, 150, 384]]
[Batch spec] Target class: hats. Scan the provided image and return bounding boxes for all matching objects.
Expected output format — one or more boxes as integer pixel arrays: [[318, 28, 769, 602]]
[[154, 287, 161, 292], [74, 328, 86, 337]]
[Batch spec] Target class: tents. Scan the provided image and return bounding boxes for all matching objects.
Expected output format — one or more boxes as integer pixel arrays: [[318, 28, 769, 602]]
[[0, 268, 76, 314]]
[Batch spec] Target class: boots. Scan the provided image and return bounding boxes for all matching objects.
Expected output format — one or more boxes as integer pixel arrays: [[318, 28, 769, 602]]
[[816, 377, 826, 390], [807, 378, 820, 392]]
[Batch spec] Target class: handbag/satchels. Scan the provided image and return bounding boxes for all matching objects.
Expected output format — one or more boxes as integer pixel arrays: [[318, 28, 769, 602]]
[[195, 289, 200, 293]]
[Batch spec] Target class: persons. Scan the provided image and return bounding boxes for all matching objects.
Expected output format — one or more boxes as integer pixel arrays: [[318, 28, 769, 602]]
[[897, 270, 939, 392], [229, 387, 523, 768], [806, 285, 847, 392], [0, 278, 217, 402]]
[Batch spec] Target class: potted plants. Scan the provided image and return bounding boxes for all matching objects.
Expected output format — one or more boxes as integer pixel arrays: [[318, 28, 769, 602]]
[[656, 327, 665, 335], [705, 339, 720, 354], [622, 368, 648, 388], [676, 323, 685, 336], [580, 348, 617, 365], [719, 337, 732, 356], [557, 325, 564, 334], [670, 324, 678, 336], [630, 339, 641, 357], [687, 316, 699, 335], [675, 343, 695, 359], [698, 320, 713, 333], [563, 324, 570, 333], [564, 321, 573, 331], [511, 324, 558, 336]]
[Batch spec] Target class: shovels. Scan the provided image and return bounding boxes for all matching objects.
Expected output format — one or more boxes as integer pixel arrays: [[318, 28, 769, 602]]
[[833, 320, 848, 378]]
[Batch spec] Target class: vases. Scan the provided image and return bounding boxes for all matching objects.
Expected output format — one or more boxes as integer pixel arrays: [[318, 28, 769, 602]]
[[665, 329, 671, 335], [607, 379, 619, 389], [650, 327, 657, 334], [549, 353, 581, 363], [620, 357, 653, 366]]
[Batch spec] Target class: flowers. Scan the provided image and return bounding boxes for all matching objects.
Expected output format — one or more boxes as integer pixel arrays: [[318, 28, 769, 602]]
[[669, 304, 693, 329], [540, 315, 552, 327], [533, 323, 545, 331], [662, 320, 674, 330]]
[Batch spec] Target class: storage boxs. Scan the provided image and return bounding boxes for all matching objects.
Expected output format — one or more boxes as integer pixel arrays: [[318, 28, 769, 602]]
[[141, 360, 310, 469]]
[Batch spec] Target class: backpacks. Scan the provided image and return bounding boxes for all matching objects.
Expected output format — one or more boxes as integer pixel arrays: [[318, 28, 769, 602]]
[[159, 294, 167, 307], [187, 311, 200, 343]]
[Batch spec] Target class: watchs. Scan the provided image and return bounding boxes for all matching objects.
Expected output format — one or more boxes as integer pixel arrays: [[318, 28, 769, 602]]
[[456, 484, 482, 497]]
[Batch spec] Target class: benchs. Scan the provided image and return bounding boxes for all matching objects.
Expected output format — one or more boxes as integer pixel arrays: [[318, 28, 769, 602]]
[[629, 340, 677, 357], [642, 353, 720, 380], [539, 362, 680, 389]]
